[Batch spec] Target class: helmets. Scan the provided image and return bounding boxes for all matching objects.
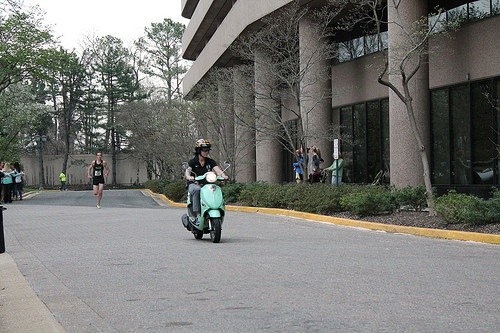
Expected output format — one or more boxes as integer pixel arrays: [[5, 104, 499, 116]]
[[194, 139, 211, 148]]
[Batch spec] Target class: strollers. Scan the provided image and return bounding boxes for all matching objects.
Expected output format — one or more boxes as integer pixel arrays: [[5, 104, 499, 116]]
[[306, 169, 327, 185]]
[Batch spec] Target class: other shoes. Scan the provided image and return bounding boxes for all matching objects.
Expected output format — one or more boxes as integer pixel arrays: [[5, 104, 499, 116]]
[[97, 204, 101, 208], [196, 215, 201, 225]]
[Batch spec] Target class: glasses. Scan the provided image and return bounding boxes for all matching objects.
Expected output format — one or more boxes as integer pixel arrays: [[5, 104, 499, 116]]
[[201, 148, 210, 152]]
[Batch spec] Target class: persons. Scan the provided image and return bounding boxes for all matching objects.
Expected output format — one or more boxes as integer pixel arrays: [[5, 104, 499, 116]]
[[88, 151, 109, 209], [59, 169, 68, 191], [0, 162, 24, 204], [293, 146, 324, 184], [185, 139, 229, 226], [324, 151, 343, 185]]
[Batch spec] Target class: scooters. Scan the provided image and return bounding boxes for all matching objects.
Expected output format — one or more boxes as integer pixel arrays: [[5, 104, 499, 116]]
[[181, 160, 231, 244]]
